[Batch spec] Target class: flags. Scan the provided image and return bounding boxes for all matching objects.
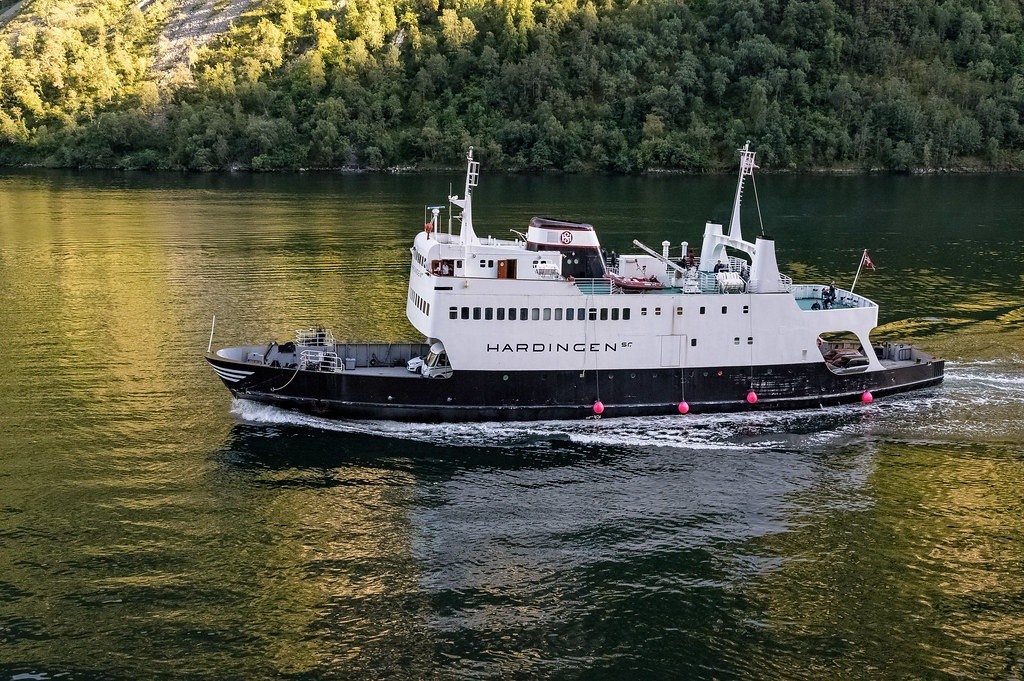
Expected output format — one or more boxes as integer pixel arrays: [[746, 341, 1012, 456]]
[[861, 249, 875, 269]]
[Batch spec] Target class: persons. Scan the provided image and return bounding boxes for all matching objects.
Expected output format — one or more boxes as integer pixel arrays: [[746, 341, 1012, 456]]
[[441, 261, 449, 276], [611, 250, 617, 267], [689, 249, 695, 266], [602, 248, 608, 261], [810, 280, 837, 310], [714, 259, 725, 286]]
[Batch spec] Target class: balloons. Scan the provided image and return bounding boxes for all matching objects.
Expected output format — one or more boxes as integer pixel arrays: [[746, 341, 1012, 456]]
[[746, 390, 758, 404], [678, 400, 690, 415], [861, 389, 874, 403], [593, 401, 605, 415]]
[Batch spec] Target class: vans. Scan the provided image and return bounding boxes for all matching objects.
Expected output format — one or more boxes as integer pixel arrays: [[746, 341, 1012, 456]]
[[420, 341, 452, 378]]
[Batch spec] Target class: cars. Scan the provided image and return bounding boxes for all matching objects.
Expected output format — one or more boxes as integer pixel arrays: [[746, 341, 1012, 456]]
[[406, 354, 427, 375], [823, 347, 870, 374]]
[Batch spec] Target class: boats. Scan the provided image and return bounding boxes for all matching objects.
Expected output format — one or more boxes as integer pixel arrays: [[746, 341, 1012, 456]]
[[602, 273, 666, 289], [202, 143, 948, 427]]
